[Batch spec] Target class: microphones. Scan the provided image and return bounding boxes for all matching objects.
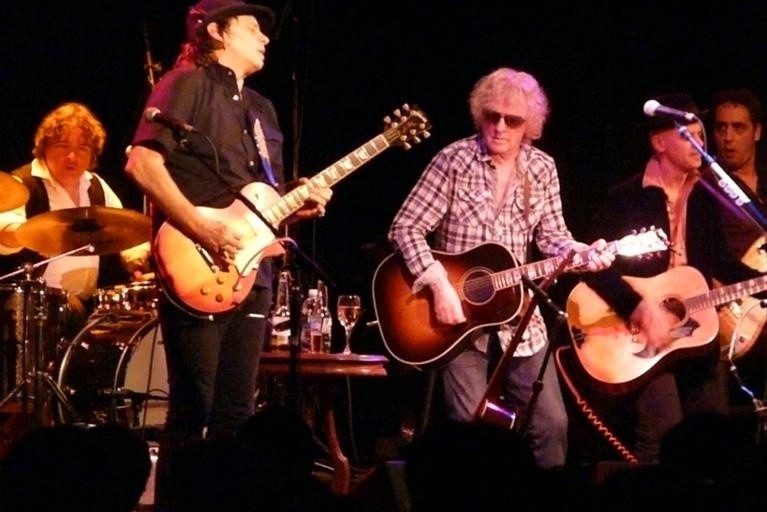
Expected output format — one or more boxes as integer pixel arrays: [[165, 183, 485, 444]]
[[644, 98, 697, 125], [519, 274, 568, 321], [140, 16, 158, 95], [282, 236, 339, 291], [141, 106, 200, 139]]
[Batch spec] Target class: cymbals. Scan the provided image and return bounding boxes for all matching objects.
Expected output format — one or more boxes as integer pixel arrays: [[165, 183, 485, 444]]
[[0, 172, 30, 213], [14, 207, 154, 256]]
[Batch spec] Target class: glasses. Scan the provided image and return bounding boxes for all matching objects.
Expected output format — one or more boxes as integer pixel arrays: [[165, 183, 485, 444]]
[[481, 106, 527, 129]]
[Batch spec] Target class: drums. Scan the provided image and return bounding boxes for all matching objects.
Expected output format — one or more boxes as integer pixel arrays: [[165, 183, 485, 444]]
[[55, 281, 169, 456]]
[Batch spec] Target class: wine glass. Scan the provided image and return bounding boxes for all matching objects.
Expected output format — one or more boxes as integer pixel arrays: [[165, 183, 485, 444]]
[[337, 293, 361, 356]]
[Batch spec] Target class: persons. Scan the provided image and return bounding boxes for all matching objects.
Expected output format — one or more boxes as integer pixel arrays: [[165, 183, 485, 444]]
[[696, 91, 767, 407], [383, 64, 617, 510], [123, 0, 334, 512], [574, 88, 738, 510], [1, 103, 123, 338], [2, 406, 767, 510]]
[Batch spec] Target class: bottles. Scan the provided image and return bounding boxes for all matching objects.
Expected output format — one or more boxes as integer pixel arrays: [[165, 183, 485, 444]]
[[270, 271, 293, 352], [299, 288, 317, 352], [309, 279, 332, 356]]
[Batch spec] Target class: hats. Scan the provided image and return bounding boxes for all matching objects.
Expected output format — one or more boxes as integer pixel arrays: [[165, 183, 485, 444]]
[[188, 0, 274, 33], [647, 95, 709, 130]]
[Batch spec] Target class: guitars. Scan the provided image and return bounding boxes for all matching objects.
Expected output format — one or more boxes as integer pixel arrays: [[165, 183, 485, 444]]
[[708, 234, 767, 362], [567, 266, 767, 395], [153, 102, 433, 318], [372, 226, 669, 372]]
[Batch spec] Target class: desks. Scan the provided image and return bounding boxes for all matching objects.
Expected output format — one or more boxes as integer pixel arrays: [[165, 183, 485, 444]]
[[257, 351, 393, 503]]
